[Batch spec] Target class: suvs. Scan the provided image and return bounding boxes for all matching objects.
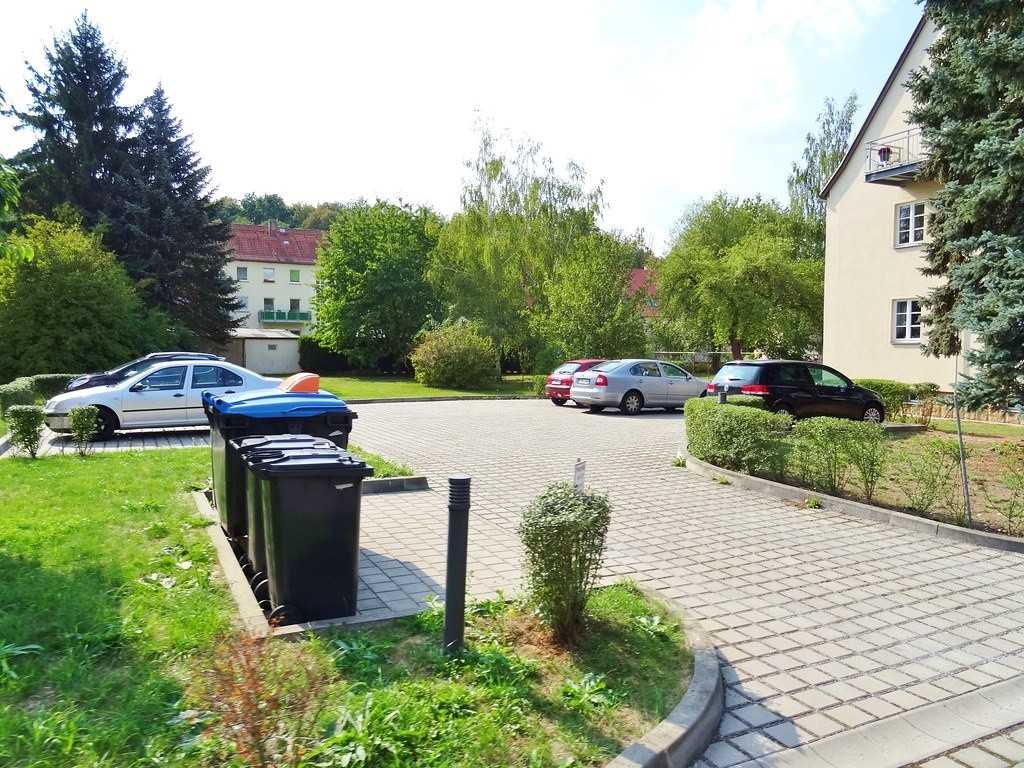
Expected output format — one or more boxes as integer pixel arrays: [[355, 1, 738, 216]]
[[706, 358, 886, 430]]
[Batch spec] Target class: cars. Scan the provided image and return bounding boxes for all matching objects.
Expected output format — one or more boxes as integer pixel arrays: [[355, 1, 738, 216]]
[[43, 360, 286, 443], [569, 359, 711, 416], [545, 359, 648, 408], [63, 352, 227, 392]]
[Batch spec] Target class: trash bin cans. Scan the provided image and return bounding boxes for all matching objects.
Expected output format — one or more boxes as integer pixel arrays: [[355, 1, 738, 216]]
[[201, 372, 358, 555], [228, 433, 375, 629]]
